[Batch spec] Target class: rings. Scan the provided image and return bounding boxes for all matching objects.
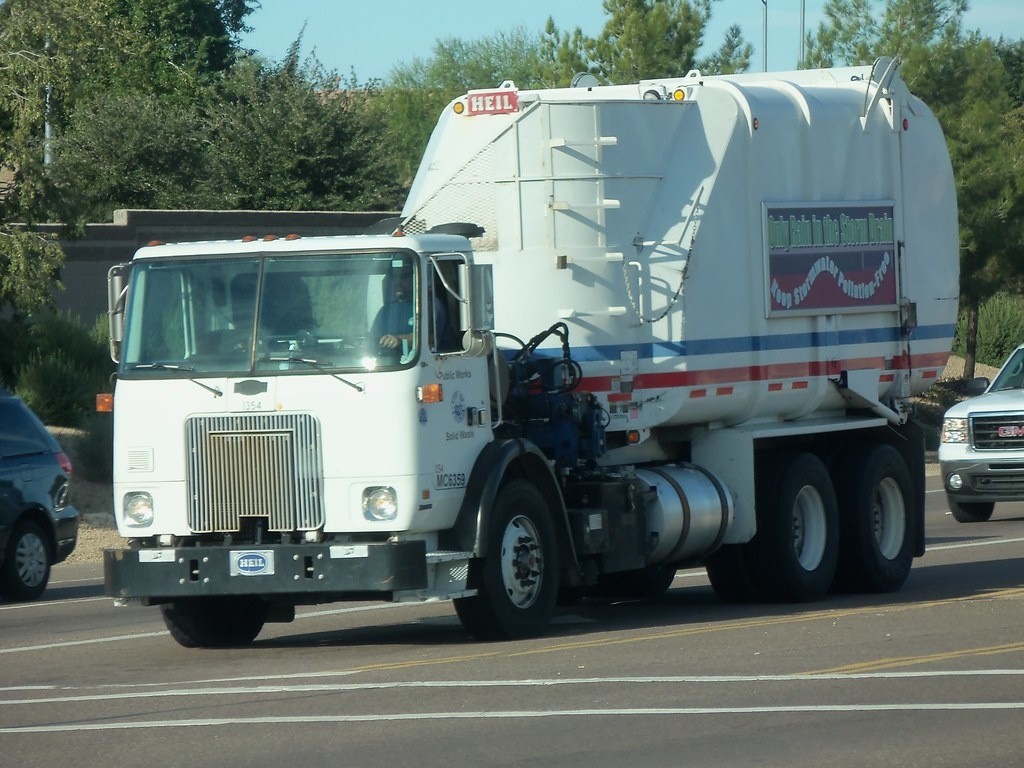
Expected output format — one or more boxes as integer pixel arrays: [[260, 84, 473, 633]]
[[390, 341, 393, 344]]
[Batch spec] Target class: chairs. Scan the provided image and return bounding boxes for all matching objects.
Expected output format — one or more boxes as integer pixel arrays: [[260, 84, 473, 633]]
[[382, 272, 460, 352], [198, 328, 270, 354]]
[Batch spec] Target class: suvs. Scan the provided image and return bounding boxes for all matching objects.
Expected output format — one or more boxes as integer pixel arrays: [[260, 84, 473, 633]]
[[0, 396, 78, 601]]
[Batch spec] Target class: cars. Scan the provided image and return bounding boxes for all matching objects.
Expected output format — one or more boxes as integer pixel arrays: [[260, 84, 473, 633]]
[[938, 343, 1024, 522]]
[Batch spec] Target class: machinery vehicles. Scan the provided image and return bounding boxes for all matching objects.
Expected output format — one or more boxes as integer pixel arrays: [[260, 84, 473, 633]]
[[96, 56, 962, 639]]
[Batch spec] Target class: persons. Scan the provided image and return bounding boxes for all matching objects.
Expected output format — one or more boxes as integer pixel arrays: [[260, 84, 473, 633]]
[[369, 265, 444, 349]]
